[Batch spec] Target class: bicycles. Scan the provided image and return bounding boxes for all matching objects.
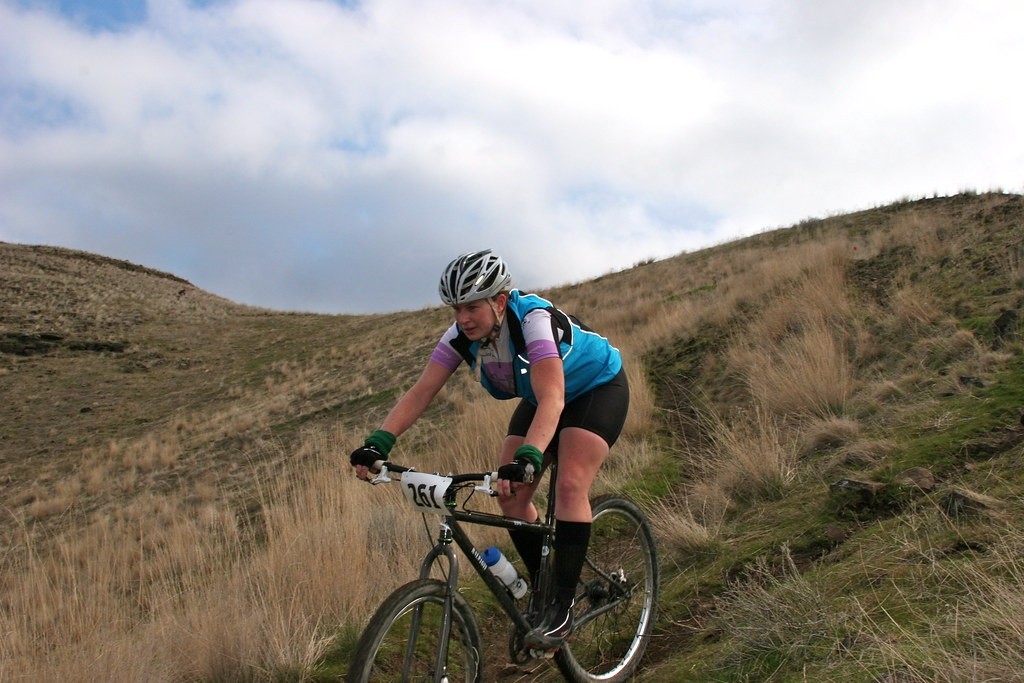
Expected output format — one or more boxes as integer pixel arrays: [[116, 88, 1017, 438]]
[[346, 459, 659, 683]]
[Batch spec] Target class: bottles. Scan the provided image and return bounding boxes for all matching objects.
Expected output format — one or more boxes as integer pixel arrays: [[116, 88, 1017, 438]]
[[481, 548, 528, 600]]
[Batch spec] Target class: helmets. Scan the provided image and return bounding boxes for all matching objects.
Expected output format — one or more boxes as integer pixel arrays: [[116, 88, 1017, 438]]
[[438, 248, 509, 306]]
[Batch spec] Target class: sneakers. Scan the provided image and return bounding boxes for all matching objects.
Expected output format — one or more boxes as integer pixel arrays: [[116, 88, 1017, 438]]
[[525, 600, 576, 650]]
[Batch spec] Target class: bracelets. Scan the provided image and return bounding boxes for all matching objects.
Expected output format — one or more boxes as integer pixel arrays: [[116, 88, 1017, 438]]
[[514, 445, 543, 477], [364, 430, 396, 454]]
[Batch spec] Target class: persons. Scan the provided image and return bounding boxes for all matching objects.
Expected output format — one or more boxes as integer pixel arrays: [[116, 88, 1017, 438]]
[[350, 249, 630, 652]]
